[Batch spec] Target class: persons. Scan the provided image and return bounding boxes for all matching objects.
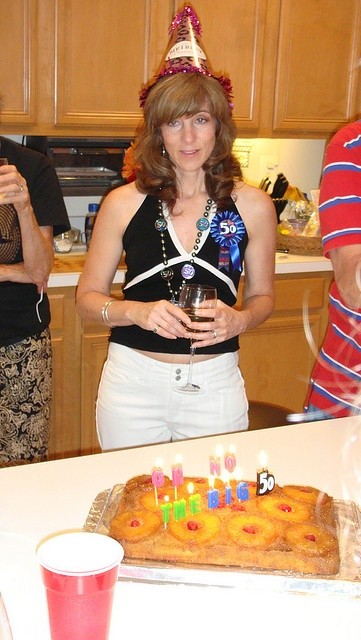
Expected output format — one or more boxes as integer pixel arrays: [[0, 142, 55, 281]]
[[0, 130, 72, 472], [300, 112, 361, 423], [75, 3, 278, 454]]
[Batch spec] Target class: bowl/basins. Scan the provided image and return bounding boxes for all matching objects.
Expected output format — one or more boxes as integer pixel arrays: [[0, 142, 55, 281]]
[[53, 237, 74, 254], [58, 227, 82, 244]]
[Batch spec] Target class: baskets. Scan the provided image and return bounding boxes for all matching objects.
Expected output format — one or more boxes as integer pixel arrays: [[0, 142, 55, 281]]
[[274, 231, 331, 254]]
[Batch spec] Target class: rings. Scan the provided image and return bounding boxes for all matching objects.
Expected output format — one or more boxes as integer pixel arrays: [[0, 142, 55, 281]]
[[17, 182, 24, 194], [150, 326, 160, 332]]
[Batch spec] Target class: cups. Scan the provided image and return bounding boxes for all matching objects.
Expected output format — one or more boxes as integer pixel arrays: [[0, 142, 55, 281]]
[[178, 285, 217, 392], [33, 532, 123, 640], [0, 157, 11, 206]]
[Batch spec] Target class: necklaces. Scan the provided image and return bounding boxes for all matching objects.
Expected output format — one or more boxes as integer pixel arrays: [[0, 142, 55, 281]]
[[156, 156, 213, 308]]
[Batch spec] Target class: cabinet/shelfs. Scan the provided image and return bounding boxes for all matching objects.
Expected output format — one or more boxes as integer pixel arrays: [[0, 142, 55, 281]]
[[273, 2, 360, 136], [80, 268, 239, 451], [54, 0, 268, 132], [47, 274, 72, 452], [1, 0, 40, 126], [239, 262, 334, 413]]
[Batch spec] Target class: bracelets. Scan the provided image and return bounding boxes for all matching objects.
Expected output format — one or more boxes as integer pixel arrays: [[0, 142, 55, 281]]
[[101, 297, 114, 327]]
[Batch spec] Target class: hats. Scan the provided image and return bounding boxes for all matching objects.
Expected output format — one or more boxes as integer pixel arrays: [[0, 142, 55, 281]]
[[139, 7, 233, 114]]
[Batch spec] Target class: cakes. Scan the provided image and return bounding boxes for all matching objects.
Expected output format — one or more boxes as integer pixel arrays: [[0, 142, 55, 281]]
[[108, 474, 341, 578]]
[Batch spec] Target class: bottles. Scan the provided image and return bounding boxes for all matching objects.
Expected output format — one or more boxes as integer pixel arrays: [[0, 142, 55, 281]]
[[84, 202, 101, 252]]
